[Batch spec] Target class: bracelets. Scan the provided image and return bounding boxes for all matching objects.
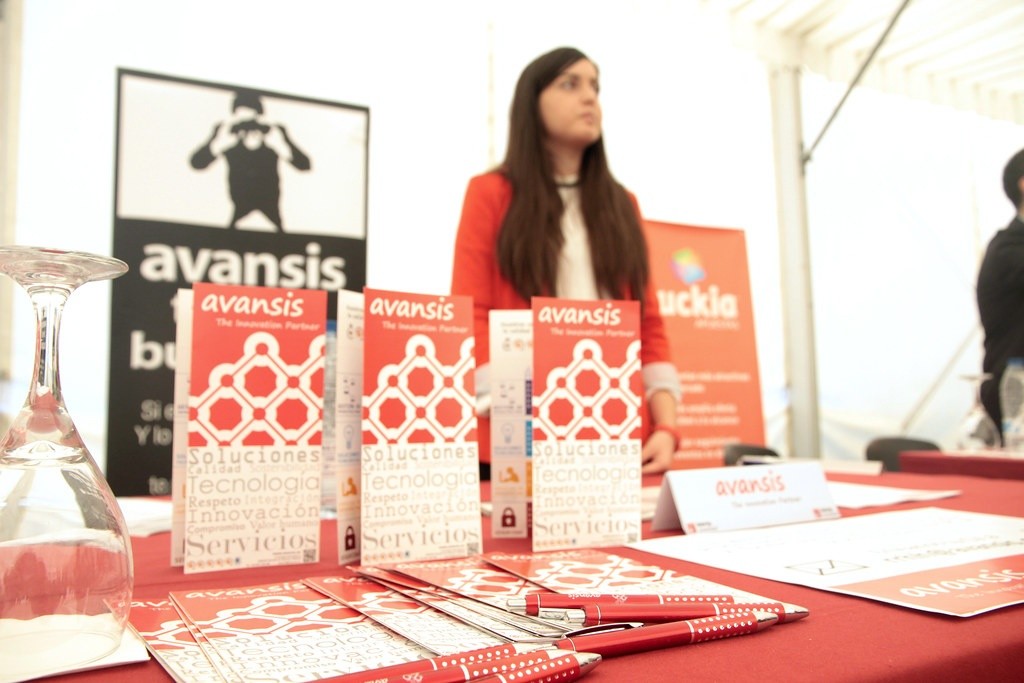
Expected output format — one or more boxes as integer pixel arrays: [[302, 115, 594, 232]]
[[652, 425, 680, 453]]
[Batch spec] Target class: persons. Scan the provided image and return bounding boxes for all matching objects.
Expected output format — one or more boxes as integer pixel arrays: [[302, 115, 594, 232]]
[[976, 149, 1024, 449], [445, 48, 681, 482]]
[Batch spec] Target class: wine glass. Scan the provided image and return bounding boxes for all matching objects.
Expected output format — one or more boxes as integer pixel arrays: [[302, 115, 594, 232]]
[[0, 245, 135, 683], [954, 374, 1001, 454]]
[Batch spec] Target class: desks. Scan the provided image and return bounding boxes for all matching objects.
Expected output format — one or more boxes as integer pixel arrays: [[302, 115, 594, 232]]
[[890, 443, 1024, 480], [0, 468, 1024, 683]]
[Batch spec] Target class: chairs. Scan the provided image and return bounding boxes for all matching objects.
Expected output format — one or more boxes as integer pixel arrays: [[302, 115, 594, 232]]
[[712, 442, 784, 469], [865, 436, 940, 468]]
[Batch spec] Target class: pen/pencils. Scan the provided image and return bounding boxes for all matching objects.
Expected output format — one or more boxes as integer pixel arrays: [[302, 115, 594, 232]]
[[506, 589, 813, 656], [304, 639, 604, 683]]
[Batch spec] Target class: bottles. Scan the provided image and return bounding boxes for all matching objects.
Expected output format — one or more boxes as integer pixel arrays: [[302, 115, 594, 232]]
[[998, 358, 1024, 457]]
